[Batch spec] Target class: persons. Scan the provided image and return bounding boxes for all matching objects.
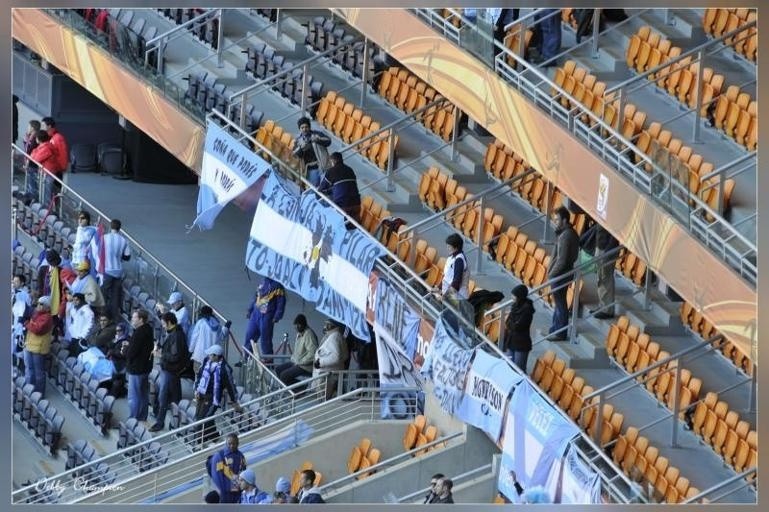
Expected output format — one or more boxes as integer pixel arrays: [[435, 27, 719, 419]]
[[462, 8, 626, 66], [11, 114, 621, 505]]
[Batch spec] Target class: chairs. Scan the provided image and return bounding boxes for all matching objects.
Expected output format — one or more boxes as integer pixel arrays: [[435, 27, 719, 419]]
[[7, 5, 762, 507]]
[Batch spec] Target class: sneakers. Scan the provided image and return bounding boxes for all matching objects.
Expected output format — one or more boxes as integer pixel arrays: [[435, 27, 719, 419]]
[[540, 330, 565, 341], [149, 422, 164, 432], [590, 309, 615, 319]]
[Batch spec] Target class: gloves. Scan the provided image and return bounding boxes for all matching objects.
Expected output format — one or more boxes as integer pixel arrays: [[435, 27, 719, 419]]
[[314, 359, 320, 370]]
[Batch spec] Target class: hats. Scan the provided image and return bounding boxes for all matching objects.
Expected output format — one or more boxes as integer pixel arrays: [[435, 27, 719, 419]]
[[38, 295, 51, 307], [204, 345, 223, 356], [276, 478, 290, 492], [75, 261, 89, 271], [167, 291, 182, 305], [240, 469, 256, 486]]
